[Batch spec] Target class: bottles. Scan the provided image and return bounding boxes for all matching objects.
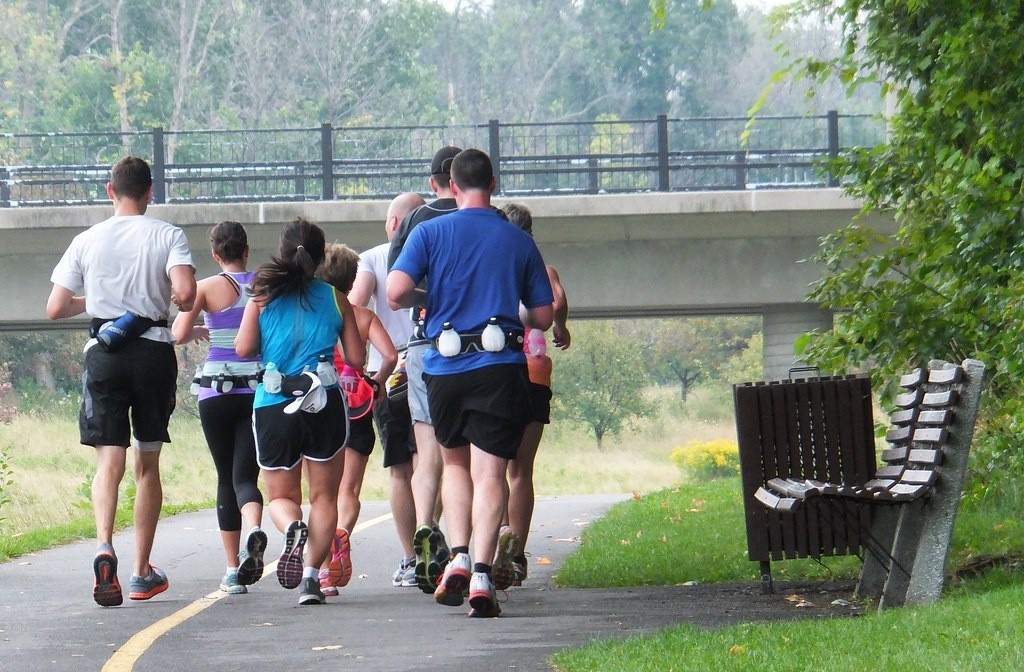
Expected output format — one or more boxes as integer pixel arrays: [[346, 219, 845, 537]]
[[248, 363, 262, 392], [339, 364, 357, 394], [528, 328, 548, 359], [438, 321, 461, 358], [189, 364, 204, 396], [317, 354, 337, 386], [263, 362, 282, 394], [412, 308, 427, 339], [96, 310, 143, 353], [212, 363, 233, 393], [481, 317, 505, 352]]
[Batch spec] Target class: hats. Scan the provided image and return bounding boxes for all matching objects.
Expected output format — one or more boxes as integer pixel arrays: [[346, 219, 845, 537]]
[[431, 147, 464, 173]]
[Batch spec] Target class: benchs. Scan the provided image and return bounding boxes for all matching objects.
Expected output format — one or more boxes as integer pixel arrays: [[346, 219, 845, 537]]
[[754, 358, 985, 610]]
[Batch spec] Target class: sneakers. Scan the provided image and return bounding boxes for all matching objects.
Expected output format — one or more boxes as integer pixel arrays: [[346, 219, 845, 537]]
[[236, 528, 267, 584], [432, 528, 451, 569], [327, 527, 352, 587], [317, 570, 340, 596], [220, 574, 247, 594], [129, 566, 168, 599], [277, 520, 308, 589], [392, 561, 418, 587], [93, 544, 123, 607], [468, 569, 502, 617], [434, 552, 471, 606], [510, 551, 531, 586], [413, 525, 444, 595], [489, 526, 519, 590], [296, 577, 326, 605]]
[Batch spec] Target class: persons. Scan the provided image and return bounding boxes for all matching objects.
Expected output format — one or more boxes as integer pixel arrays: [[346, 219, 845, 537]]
[[46, 155, 197, 606], [387, 147, 463, 595], [350, 192, 451, 587], [386, 148, 554, 617], [314, 244, 398, 596], [491, 201, 571, 592], [171, 221, 267, 593], [234, 216, 366, 605]]
